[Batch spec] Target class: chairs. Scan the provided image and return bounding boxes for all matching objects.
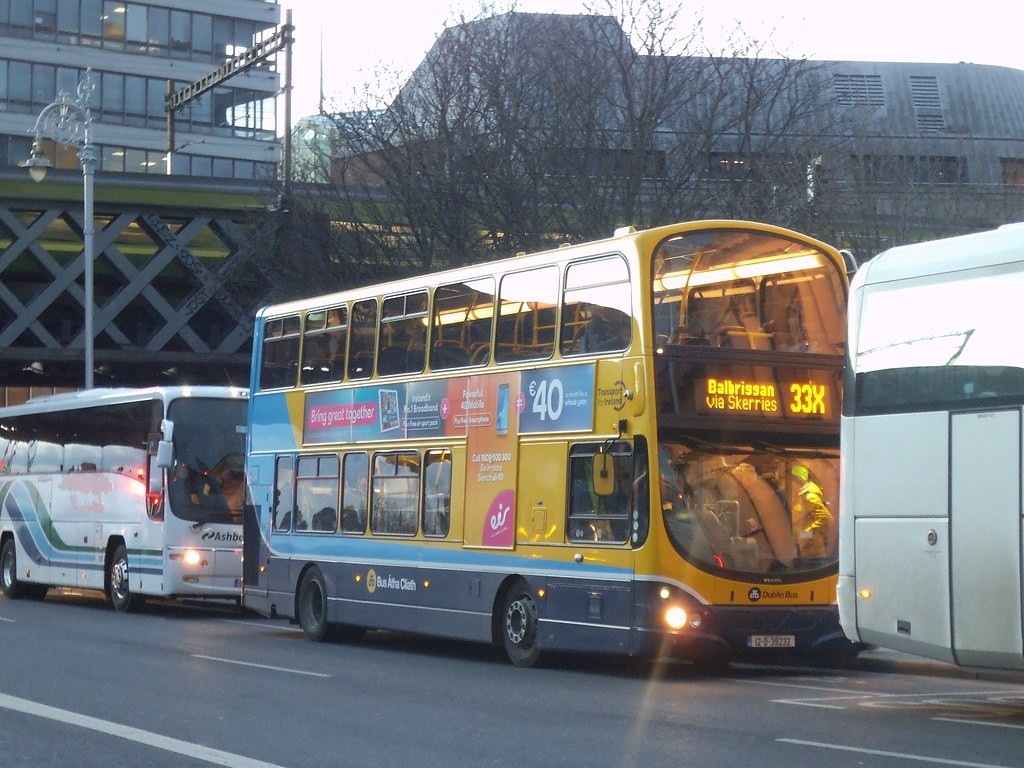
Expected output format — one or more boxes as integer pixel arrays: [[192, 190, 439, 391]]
[[655, 325, 791, 353], [262, 337, 534, 389]]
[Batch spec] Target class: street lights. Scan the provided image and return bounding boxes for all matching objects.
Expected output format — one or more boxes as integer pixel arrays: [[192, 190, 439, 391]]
[[20, 66, 98, 389]]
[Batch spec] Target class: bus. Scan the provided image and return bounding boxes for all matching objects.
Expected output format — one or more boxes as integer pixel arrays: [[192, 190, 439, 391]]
[[243, 218, 858, 674], [834, 220, 1022, 675], [1, 385, 250, 613]]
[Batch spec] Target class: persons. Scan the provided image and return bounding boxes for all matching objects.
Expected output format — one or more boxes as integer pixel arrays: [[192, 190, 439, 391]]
[[588, 303, 631, 355], [773, 307, 809, 352], [689, 296, 712, 339], [787, 465, 831, 560], [356, 474, 380, 533]]
